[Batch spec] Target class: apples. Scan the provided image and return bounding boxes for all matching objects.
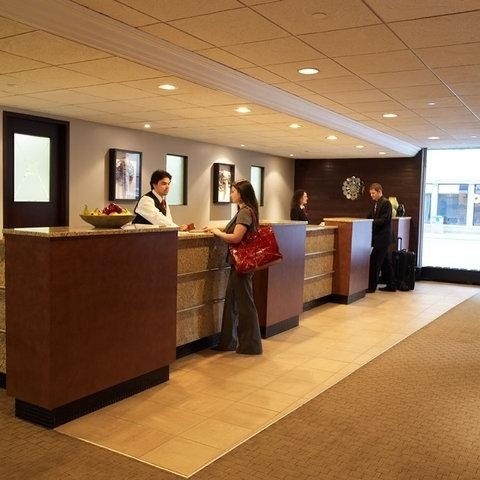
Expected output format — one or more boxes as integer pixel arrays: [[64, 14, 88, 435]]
[[101, 203, 123, 216]]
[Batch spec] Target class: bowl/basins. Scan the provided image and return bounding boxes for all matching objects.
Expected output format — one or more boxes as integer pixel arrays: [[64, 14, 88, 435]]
[[80, 214, 138, 228]]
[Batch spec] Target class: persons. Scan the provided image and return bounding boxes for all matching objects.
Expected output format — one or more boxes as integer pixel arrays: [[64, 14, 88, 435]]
[[203, 180, 263, 355], [290, 190, 312, 225], [132, 170, 188, 232], [366, 183, 397, 293]]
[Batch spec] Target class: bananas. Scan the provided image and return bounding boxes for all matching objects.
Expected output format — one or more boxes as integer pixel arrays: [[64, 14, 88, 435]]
[[82, 205, 101, 216], [113, 208, 131, 215]]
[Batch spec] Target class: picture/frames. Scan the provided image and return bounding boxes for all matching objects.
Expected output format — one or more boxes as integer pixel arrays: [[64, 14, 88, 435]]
[[109, 147, 142, 202], [213, 163, 235, 204]]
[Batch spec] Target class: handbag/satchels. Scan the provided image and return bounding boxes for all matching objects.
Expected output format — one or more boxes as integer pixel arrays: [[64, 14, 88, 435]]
[[228, 207, 283, 275]]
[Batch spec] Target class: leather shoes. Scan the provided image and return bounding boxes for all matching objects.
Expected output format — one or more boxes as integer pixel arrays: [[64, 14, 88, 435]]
[[379, 287, 396, 292], [366, 289, 375, 293]]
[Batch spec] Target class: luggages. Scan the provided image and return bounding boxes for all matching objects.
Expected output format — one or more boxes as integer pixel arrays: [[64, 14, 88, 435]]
[[392, 236, 418, 292]]
